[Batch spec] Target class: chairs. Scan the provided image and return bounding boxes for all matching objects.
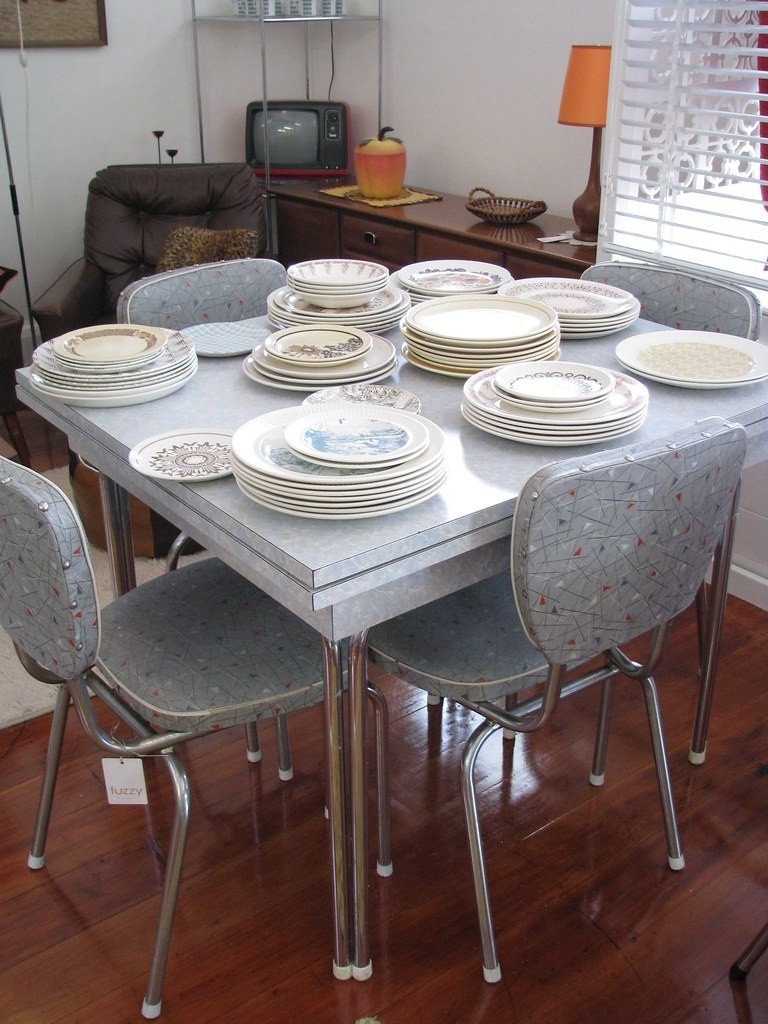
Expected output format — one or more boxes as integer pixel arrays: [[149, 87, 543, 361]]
[[498, 262, 762, 765], [1, 455, 397, 1021], [360, 419, 748, 981], [118, 256, 266, 764]]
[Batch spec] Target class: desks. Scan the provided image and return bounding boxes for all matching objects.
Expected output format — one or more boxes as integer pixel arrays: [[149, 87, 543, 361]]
[[15, 313, 767, 981]]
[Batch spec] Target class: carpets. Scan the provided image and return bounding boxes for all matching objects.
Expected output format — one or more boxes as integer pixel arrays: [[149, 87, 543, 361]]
[[0, 463, 216, 731]]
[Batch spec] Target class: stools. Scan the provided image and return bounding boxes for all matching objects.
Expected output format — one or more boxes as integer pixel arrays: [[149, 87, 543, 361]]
[[70, 452, 208, 559]]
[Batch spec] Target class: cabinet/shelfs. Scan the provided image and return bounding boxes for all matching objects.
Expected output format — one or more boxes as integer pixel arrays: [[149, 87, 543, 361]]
[[264, 176, 598, 280]]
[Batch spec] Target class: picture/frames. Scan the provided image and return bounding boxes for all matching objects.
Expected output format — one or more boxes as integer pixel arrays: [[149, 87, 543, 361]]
[[0, 1, 108, 47]]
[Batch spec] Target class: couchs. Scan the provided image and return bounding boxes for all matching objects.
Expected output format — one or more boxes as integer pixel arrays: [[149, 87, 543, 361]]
[[31, 162, 268, 343]]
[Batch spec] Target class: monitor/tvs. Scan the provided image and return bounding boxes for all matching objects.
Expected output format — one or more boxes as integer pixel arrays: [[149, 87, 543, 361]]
[[243, 101, 350, 175]]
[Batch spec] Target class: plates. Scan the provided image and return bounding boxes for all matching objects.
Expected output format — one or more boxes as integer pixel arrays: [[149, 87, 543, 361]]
[[28, 324, 199, 407], [391, 259, 768, 446], [243, 257, 411, 392], [129, 386, 447, 519]]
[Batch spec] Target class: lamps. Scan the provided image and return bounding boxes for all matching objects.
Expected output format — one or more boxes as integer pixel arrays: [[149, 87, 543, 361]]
[[557, 45, 613, 242]]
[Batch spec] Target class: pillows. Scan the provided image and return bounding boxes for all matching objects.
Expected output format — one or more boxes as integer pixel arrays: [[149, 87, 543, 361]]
[[155, 225, 257, 272]]
